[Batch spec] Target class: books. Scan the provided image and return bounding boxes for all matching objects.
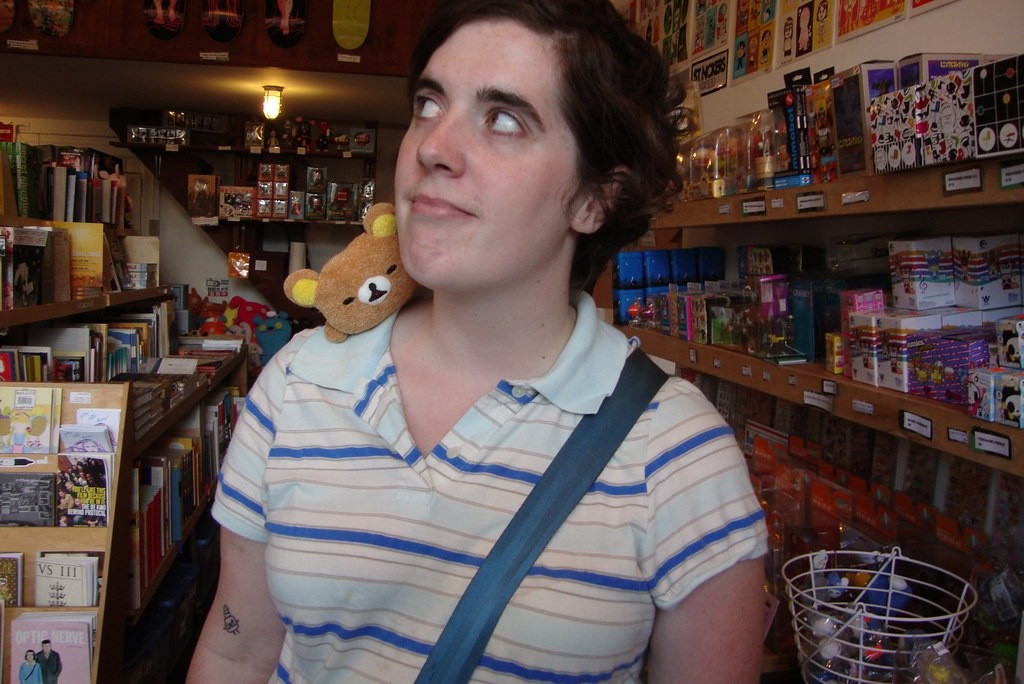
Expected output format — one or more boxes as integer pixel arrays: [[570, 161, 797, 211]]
[[1, 124, 247, 684]]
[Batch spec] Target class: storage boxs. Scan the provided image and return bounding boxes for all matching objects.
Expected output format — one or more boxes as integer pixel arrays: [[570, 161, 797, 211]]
[[0, 52, 1024, 684]]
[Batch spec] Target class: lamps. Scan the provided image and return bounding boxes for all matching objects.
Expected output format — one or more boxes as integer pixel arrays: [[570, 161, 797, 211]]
[[262, 85, 285, 120]]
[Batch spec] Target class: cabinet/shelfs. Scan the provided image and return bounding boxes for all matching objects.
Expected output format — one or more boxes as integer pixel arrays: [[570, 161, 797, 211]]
[[599, 160, 1024, 674], [0, 147, 251, 684], [109, 115, 245, 252], [239, 119, 378, 253]]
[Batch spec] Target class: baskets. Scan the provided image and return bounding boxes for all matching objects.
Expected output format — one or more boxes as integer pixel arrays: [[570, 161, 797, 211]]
[[781, 546, 979, 684]]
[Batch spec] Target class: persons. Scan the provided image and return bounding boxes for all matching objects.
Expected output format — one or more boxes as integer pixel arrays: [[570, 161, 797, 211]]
[[54, 460, 108, 527], [19, 649, 43, 684], [37, 639, 63, 684], [184, 1, 770, 684], [80, 438, 105, 453]]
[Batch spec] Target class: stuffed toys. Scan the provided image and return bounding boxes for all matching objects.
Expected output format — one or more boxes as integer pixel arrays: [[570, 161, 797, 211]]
[[283, 201, 420, 344]]
